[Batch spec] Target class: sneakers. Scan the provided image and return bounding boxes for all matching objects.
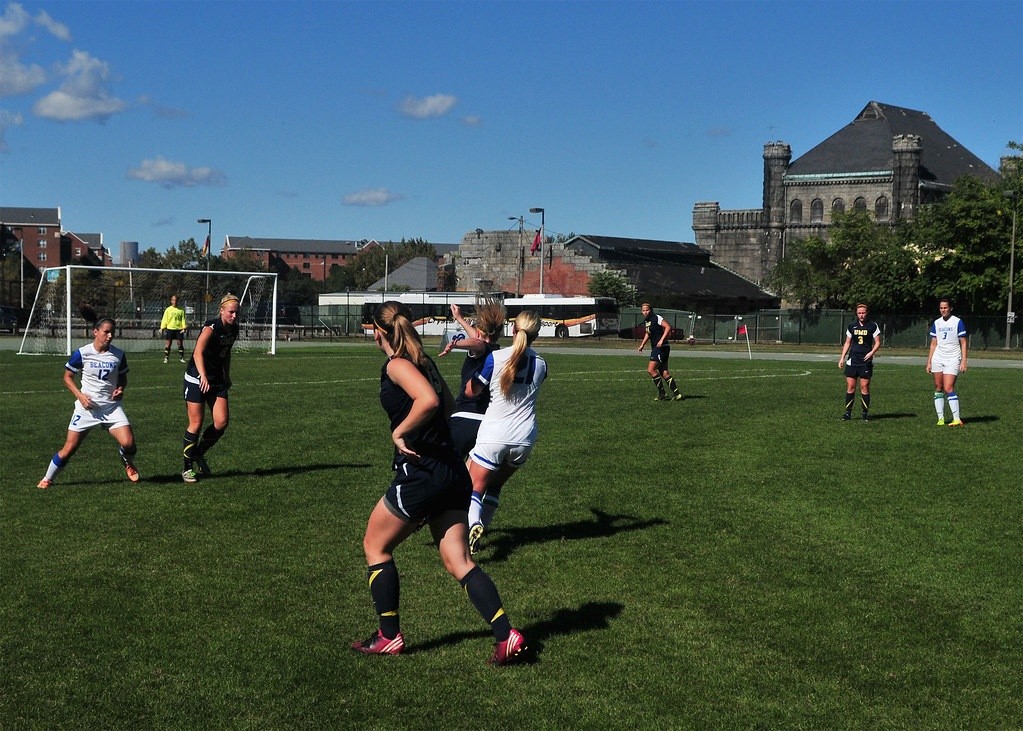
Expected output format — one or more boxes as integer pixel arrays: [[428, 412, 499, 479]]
[[196, 454, 211, 478], [180, 358, 186, 364], [487, 628, 528, 668], [37, 479, 52, 490], [840, 413, 851, 420], [862, 416, 871, 422], [353, 629, 406, 655], [124, 460, 140, 482], [937, 419, 945, 426], [670, 393, 682, 402], [948, 419, 964, 426], [467, 521, 484, 555], [652, 393, 668, 402], [182, 469, 197, 483], [164, 357, 169, 363]]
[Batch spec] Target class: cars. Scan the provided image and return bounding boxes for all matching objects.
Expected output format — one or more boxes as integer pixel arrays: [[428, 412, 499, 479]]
[[619, 321, 684, 340]]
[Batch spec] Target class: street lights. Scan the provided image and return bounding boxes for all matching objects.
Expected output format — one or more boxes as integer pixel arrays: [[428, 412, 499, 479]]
[[530, 207, 544, 293], [197, 219, 212, 322], [507, 217, 523, 298]]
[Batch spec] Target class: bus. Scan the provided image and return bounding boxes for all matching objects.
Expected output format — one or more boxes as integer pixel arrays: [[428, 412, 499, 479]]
[[362, 292, 619, 339]]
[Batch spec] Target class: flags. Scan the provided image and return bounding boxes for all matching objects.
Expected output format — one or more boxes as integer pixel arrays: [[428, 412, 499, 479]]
[[531, 230, 541, 255]]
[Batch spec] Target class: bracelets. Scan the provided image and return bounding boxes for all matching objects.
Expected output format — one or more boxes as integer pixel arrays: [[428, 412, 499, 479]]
[[452, 340, 457, 348]]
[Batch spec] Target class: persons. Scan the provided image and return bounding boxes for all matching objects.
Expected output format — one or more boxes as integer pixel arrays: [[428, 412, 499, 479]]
[[37, 306, 140, 489], [159, 296, 187, 364], [838, 303, 881, 422], [927, 299, 967, 426], [638, 301, 684, 401], [182, 293, 241, 483], [350, 300, 529, 665], [413, 304, 548, 554]]
